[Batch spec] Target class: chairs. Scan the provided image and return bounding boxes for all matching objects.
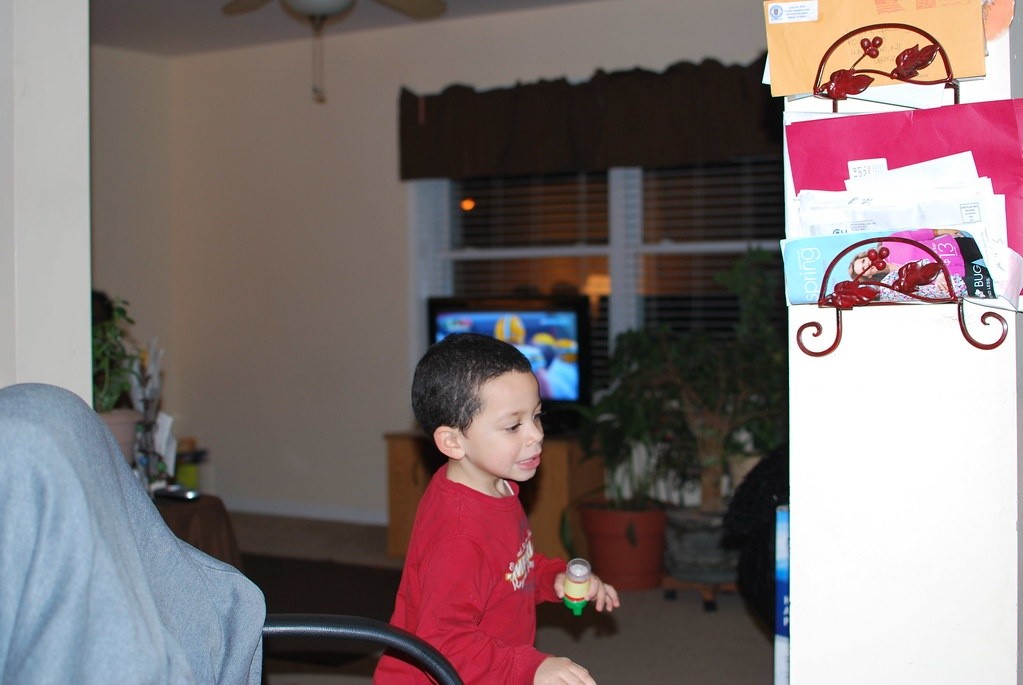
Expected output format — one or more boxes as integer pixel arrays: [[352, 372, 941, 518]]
[[0, 382, 463, 685]]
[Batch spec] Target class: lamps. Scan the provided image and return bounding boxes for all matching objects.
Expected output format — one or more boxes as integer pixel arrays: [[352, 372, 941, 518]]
[[279, 0, 353, 105]]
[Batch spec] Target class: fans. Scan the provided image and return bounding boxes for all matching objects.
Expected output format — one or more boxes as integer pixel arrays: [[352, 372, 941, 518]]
[[223, 0, 447, 21]]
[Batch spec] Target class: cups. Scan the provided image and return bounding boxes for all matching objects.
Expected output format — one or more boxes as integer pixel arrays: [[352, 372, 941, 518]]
[[563, 558, 590, 615]]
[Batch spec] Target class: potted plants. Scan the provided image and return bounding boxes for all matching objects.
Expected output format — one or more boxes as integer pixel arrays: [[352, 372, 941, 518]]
[[91, 287, 146, 468], [573, 326, 702, 590]]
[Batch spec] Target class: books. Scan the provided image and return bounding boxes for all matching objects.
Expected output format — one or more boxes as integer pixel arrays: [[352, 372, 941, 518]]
[[780, 220, 997, 299]]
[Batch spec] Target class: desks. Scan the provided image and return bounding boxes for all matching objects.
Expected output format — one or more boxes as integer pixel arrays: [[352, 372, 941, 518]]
[[155, 493, 241, 569]]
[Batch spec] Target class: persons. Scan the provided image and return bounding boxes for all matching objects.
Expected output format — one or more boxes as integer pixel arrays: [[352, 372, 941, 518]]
[[373, 332, 620, 685], [848, 228, 994, 288]]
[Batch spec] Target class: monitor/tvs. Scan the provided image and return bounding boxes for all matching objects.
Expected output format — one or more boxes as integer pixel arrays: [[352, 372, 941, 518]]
[[428, 296, 592, 439]]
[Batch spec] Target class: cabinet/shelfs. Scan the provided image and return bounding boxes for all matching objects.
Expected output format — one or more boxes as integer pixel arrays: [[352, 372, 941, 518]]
[[382, 429, 606, 562]]
